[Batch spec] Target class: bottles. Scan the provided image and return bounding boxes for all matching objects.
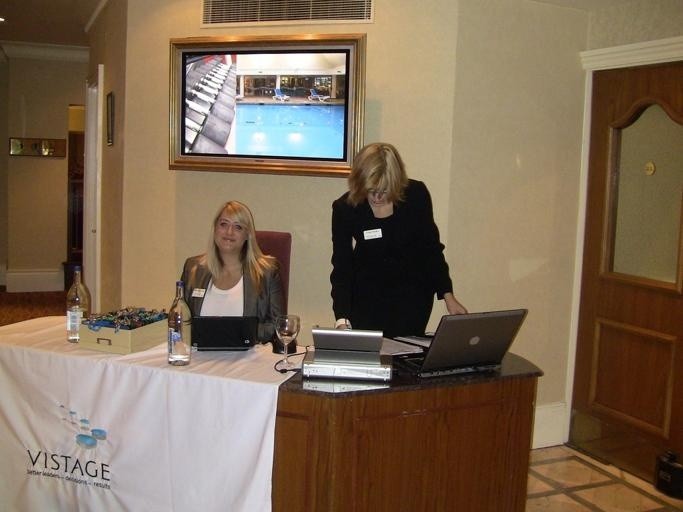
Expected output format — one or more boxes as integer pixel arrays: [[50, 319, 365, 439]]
[[168, 280, 192, 366], [66, 265, 93, 345]]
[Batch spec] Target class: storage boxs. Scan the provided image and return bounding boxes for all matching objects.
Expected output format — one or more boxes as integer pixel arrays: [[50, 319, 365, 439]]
[[78, 317, 168, 355]]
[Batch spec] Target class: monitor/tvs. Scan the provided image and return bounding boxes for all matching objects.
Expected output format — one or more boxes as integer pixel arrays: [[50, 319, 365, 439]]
[[181, 49, 350, 161]]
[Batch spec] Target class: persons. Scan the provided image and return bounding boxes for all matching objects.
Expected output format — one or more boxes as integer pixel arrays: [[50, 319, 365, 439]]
[[328, 143, 467, 340], [171, 199, 286, 343]]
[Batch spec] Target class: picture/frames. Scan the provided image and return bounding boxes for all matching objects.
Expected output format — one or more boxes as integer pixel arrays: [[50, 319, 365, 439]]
[[107, 91, 114, 146], [168, 32, 366, 180]]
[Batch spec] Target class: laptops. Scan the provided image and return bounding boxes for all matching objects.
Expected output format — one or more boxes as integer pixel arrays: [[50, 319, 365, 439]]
[[392, 309, 525, 379], [190, 316, 258, 351]]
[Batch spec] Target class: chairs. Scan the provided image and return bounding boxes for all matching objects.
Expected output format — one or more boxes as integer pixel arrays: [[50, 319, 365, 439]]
[[255, 232, 292, 315]]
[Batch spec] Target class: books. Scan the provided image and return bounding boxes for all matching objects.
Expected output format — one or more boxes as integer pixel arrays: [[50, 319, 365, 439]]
[[379, 337, 424, 357], [393, 335, 432, 348]]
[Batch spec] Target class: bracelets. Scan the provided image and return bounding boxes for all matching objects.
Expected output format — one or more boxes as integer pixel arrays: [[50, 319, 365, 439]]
[[333, 317, 352, 329]]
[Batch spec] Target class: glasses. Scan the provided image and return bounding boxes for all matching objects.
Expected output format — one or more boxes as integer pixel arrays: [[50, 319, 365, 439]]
[[368, 188, 388, 193]]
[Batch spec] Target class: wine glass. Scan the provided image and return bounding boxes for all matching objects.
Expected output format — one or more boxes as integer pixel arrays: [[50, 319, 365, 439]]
[[274, 315, 300, 369]]
